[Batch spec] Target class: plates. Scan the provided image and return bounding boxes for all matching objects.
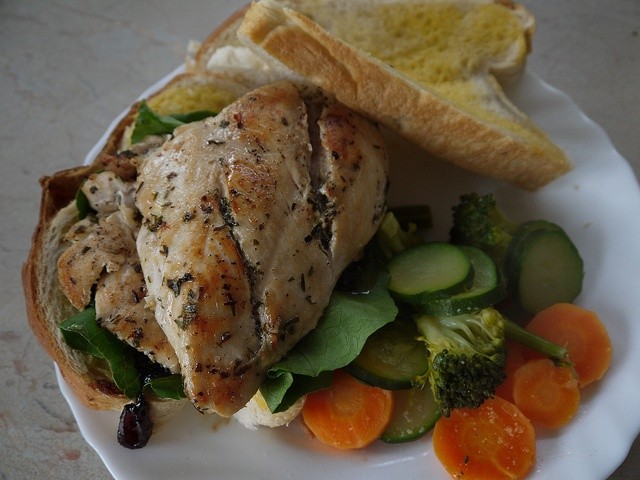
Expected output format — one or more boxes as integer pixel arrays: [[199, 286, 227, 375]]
[[52, 63, 640, 479]]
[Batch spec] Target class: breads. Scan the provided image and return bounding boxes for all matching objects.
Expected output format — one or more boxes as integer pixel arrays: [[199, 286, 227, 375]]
[[97, 4, 290, 160], [234, 0, 576, 195], [21, 148, 187, 417]]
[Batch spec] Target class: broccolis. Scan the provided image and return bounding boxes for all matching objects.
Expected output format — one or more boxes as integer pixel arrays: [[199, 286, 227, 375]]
[[409, 308, 516, 420]]
